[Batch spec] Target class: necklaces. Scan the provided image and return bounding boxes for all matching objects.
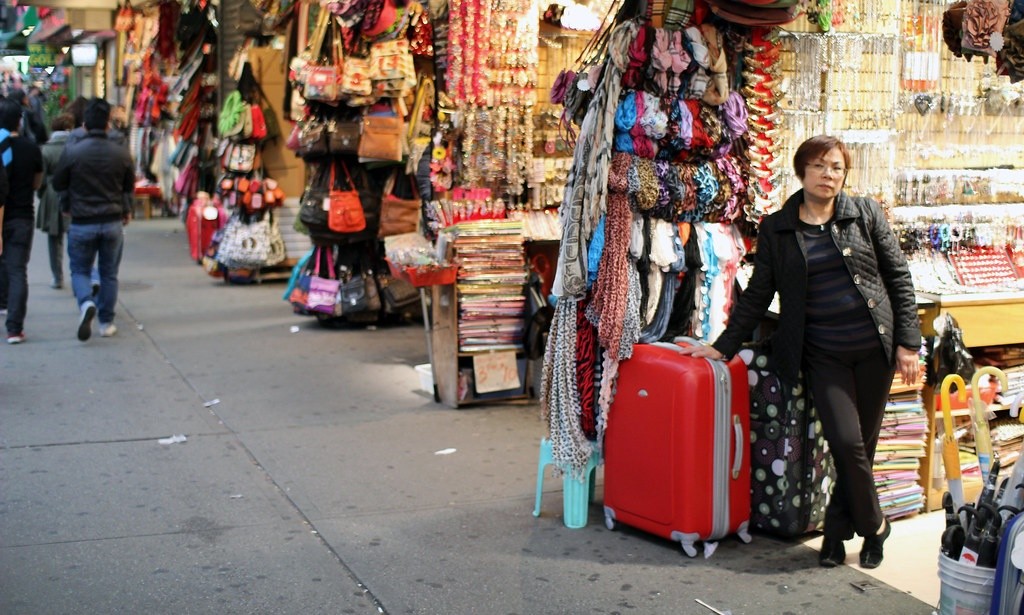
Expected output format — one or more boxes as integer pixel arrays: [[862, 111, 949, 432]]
[[809, 214, 831, 231]]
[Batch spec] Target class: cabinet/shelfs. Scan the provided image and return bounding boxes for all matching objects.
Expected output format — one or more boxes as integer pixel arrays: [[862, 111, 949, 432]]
[[431, 221, 532, 409], [922, 292, 1024, 511]]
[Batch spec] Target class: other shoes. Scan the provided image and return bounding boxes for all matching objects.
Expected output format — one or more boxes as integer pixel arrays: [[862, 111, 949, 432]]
[[6, 332, 24, 343], [820, 536, 845, 566], [100, 325, 116, 336], [91, 278, 101, 298], [860, 518, 890, 567], [51, 281, 63, 288], [78, 300, 96, 340]]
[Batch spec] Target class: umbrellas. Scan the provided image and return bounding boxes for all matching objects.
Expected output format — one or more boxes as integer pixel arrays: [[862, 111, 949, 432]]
[[940, 374, 967, 532], [968, 367, 1009, 487], [942, 389, 1024, 569]]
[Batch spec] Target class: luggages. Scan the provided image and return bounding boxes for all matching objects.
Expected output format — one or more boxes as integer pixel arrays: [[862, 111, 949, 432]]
[[740, 343, 835, 537], [188, 199, 219, 260], [603, 338, 751, 554]]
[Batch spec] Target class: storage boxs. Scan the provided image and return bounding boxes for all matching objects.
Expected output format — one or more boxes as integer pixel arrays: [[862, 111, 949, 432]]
[[383, 256, 458, 285]]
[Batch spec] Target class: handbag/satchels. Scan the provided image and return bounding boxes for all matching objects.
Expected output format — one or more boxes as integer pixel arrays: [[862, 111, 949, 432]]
[[564, 73, 594, 125], [213, 0, 422, 317], [551, 68, 575, 104]]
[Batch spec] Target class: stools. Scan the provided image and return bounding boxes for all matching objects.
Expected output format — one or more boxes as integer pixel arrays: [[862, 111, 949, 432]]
[[531, 437, 602, 530], [131, 193, 152, 218]]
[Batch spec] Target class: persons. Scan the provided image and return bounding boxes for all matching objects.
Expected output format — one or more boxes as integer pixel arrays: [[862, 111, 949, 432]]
[[0, 68, 130, 343], [52, 97, 134, 342], [678, 133, 921, 569]]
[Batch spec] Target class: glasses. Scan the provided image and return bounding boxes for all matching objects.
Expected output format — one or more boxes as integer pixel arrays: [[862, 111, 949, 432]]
[[806, 162, 848, 177]]
